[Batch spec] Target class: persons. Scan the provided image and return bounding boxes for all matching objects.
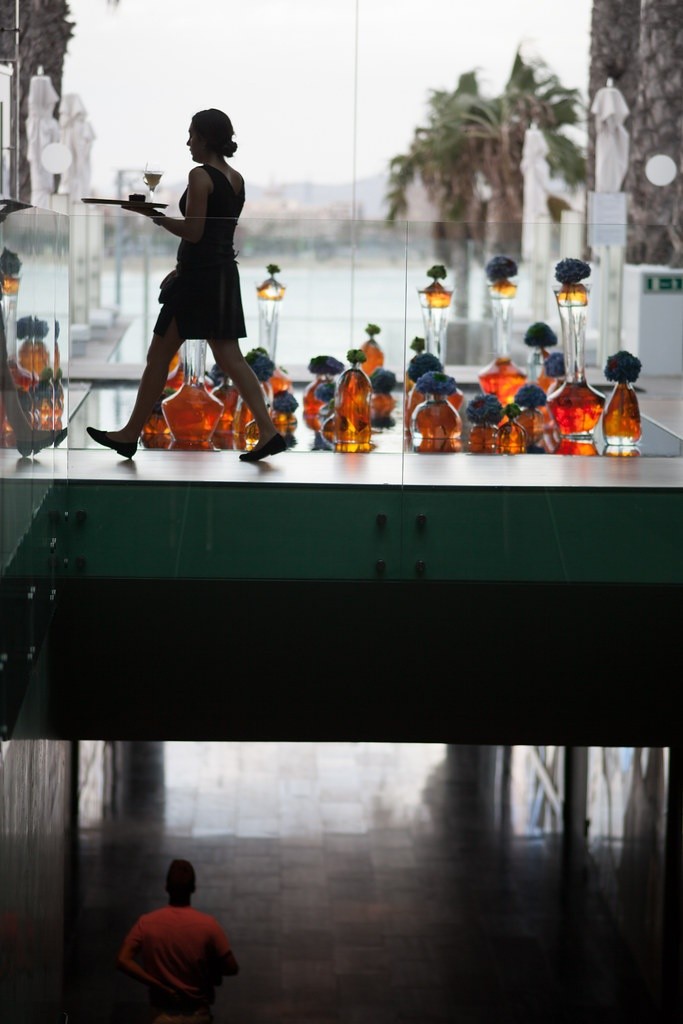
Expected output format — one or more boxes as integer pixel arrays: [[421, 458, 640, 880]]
[[118, 858, 240, 1024], [1, 199, 68, 461], [85, 109, 288, 462]]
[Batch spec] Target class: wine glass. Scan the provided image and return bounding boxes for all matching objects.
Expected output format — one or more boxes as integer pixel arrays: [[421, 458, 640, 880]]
[[143, 170, 163, 203]]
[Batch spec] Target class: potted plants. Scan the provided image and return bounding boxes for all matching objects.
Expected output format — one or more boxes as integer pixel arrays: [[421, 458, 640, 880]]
[[379, 36, 594, 273]]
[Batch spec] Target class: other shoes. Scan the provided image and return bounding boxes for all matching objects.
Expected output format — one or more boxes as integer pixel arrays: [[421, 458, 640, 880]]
[[16, 427, 67, 457], [239, 433, 287, 461], [86, 426, 138, 459]]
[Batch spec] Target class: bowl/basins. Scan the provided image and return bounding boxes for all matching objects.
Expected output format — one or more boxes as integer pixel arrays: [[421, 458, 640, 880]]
[[128, 193, 147, 202]]
